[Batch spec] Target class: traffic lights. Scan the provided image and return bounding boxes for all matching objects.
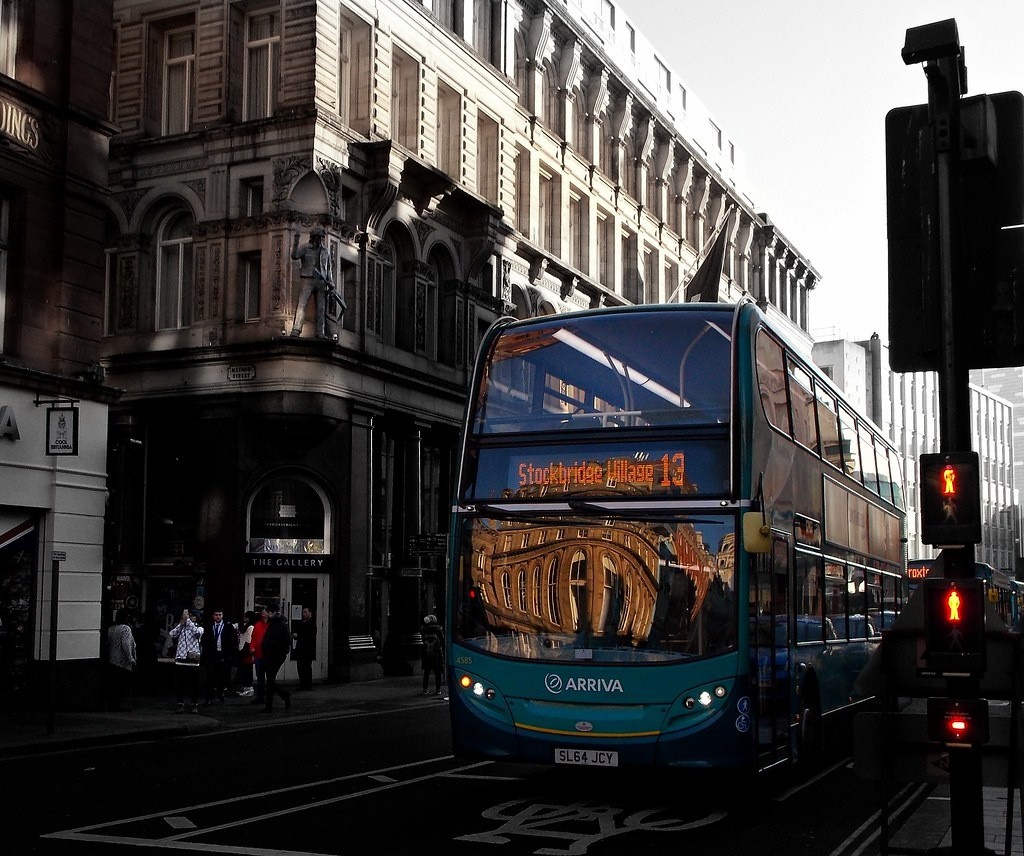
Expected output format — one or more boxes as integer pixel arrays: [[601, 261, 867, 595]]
[[920, 453, 984, 546], [922, 577, 989, 662], [926, 696, 990, 745]]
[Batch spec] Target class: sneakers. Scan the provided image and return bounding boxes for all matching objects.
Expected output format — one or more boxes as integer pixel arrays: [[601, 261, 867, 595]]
[[423, 689, 430, 695], [436, 688, 443, 695], [174, 702, 186, 714], [188, 700, 200, 714], [236, 686, 254, 696]]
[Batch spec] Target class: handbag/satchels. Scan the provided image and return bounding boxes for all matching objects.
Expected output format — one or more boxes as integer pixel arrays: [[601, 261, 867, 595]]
[[186, 651, 201, 663], [274, 645, 298, 686]]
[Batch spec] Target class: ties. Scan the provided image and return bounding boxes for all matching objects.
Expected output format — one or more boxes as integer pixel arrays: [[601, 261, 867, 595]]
[[215, 624, 220, 644]]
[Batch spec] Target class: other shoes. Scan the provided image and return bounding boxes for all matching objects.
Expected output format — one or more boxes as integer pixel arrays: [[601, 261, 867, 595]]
[[285, 692, 292, 713], [257, 705, 273, 715]]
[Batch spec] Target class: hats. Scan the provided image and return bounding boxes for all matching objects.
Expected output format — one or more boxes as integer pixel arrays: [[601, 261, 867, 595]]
[[423, 615, 437, 625], [189, 608, 201, 619]]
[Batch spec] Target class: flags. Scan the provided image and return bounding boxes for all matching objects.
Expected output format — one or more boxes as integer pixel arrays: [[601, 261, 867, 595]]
[[683, 212, 730, 303]]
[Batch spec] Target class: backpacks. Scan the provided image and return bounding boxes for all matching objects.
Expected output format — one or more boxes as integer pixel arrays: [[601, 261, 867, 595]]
[[423, 627, 440, 659]]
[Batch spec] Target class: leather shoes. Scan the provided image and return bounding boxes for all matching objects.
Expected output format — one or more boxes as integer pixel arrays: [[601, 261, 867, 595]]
[[218, 689, 225, 703], [203, 698, 214, 707]]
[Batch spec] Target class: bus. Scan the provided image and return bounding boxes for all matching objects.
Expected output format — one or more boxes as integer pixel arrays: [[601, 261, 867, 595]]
[[904, 560, 1012, 628], [436, 295, 911, 807]]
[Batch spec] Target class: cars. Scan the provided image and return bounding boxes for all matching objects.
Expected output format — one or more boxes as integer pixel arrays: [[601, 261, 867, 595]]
[[745, 603, 908, 715]]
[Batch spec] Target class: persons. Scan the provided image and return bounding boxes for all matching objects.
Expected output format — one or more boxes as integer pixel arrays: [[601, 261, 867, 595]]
[[258, 602, 292, 714], [295, 607, 316, 690], [236, 610, 255, 697], [653, 571, 711, 650], [206, 607, 237, 705], [106, 609, 139, 712], [250, 606, 270, 704], [169, 608, 204, 714], [800, 518, 820, 547], [420, 614, 446, 694]]
